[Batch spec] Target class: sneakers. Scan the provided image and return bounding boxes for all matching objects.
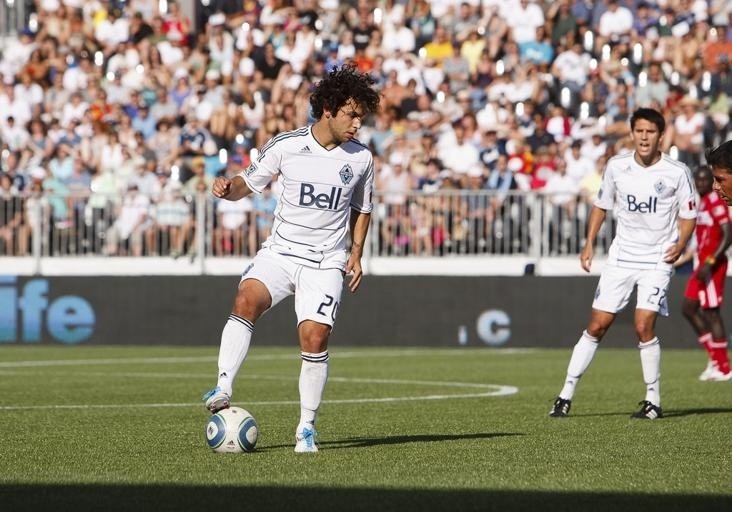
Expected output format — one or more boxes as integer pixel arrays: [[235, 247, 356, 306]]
[[699, 360, 732, 381], [201, 387, 230, 413], [295, 425, 318, 453], [630, 400, 663, 420], [549, 397, 571, 417]]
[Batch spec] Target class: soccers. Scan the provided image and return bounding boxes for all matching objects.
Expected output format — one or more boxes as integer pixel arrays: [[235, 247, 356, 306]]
[[206, 406, 258, 454]]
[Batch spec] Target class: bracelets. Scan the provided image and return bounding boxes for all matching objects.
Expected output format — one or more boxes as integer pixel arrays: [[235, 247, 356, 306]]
[[704, 256, 716, 265]]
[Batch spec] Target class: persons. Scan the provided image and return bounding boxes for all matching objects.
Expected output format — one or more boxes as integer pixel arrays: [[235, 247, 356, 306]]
[[680, 166, 732, 384], [545, 107, 701, 423], [1, 0, 732, 259], [199, 62, 386, 455]]
